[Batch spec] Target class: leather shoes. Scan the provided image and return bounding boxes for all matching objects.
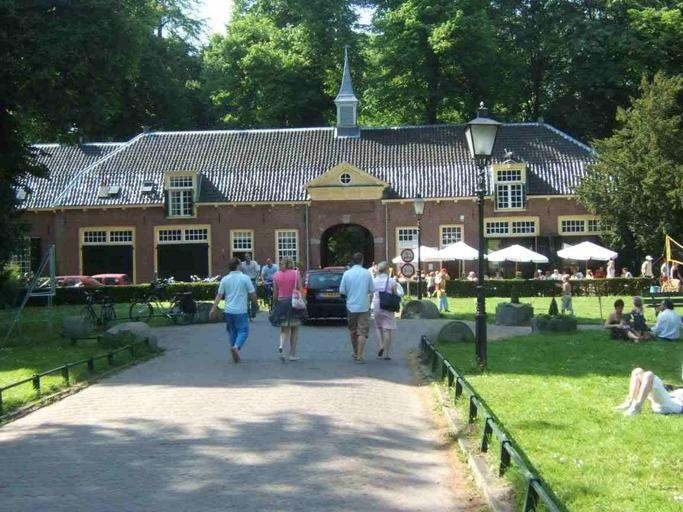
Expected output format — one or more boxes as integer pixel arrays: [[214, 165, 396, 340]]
[[278, 347, 286, 362], [289, 355, 301, 361]]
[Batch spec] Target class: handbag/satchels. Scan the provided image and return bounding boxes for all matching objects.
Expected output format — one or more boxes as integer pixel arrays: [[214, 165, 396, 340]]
[[379, 275, 400, 313], [292, 269, 306, 311]]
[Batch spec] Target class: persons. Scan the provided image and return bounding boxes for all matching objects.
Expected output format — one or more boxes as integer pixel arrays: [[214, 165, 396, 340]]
[[368, 262, 477, 313], [207, 256, 258, 364], [371, 261, 404, 361], [534, 256, 681, 279], [609, 362, 682, 418], [604, 296, 680, 341], [338, 252, 375, 362], [562, 273, 574, 317], [266, 255, 308, 364], [241, 252, 261, 324], [260, 254, 277, 305]]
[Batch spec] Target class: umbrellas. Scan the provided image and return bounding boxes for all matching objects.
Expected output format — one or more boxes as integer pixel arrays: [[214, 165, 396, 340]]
[[439, 241, 487, 280], [555, 241, 619, 277], [390, 245, 454, 277], [485, 241, 550, 278]]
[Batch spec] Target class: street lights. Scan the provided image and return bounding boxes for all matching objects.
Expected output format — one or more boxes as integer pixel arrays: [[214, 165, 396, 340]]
[[462, 99, 505, 372], [412, 190, 428, 301], [15, 185, 27, 279]]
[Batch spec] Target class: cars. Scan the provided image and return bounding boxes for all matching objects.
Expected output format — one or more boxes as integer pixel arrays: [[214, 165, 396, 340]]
[[31, 272, 131, 289], [301, 266, 348, 322]]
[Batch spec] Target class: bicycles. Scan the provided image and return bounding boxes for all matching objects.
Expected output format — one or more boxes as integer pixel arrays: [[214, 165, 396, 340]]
[[77, 278, 199, 330], [244, 273, 274, 323]]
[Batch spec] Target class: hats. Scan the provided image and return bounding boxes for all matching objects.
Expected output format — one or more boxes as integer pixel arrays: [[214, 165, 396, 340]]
[[645, 255, 653, 261]]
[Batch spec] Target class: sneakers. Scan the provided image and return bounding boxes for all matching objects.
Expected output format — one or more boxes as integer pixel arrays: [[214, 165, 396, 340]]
[[231, 347, 240, 361], [384, 356, 392, 360], [378, 347, 384, 357], [614, 399, 641, 415], [351, 353, 365, 364]]
